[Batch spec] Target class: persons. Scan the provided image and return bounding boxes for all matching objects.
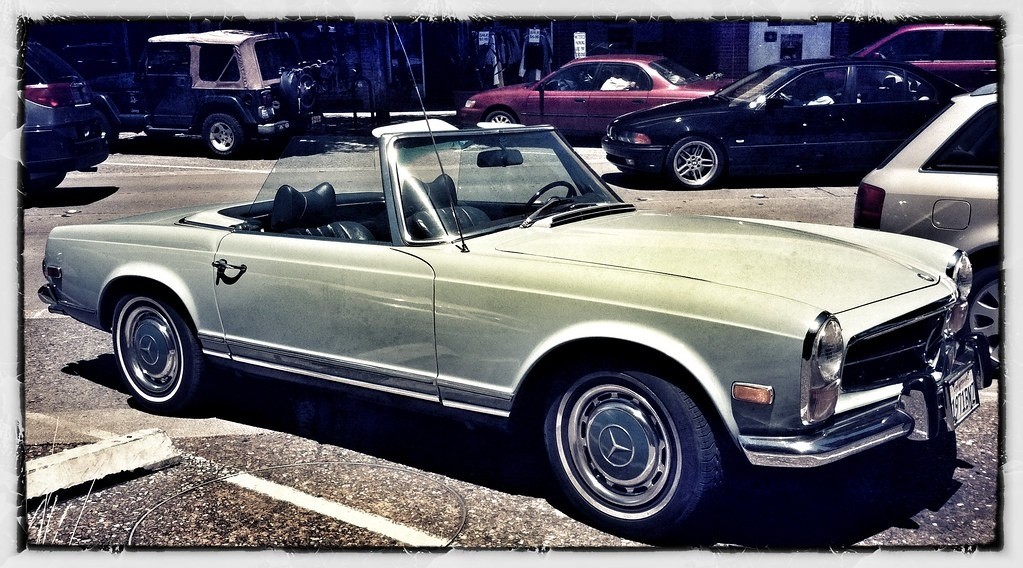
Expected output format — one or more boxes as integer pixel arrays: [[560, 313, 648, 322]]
[[790, 73, 833, 105]]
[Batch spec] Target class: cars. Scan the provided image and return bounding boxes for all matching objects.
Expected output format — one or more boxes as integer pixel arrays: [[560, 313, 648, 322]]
[[853, 80, 1003, 377], [37, 125, 982, 543], [836, 24, 999, 91], [460, 53, 740, 141], [603, 58, 971, 190]]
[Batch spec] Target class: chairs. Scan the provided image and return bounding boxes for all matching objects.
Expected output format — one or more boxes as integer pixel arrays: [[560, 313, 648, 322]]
[[402, 174, 491, 240], [882, 77, 896, 89], [272, 184, 372, 241]]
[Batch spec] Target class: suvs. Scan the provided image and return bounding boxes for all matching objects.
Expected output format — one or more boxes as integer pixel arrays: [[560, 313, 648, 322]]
[[23, 39, 123, 203], [75, 30, 320, 157]]
